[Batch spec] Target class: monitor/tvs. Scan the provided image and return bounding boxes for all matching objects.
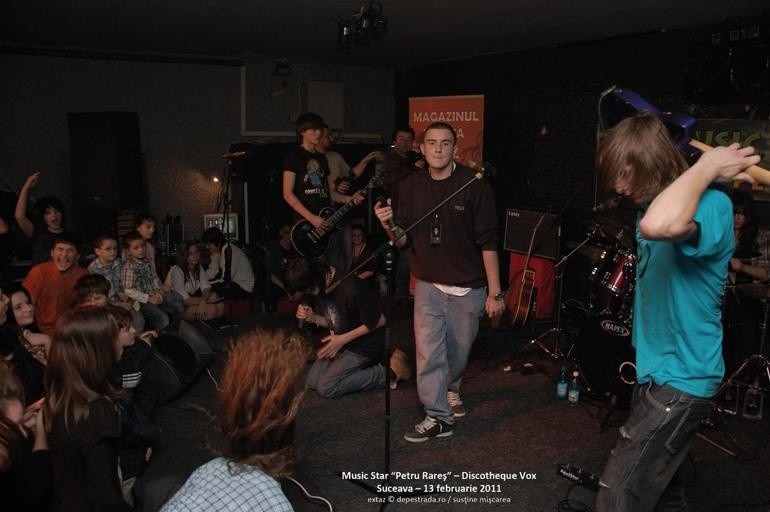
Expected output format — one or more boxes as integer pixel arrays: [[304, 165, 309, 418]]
[[205, 215, 238, 242]]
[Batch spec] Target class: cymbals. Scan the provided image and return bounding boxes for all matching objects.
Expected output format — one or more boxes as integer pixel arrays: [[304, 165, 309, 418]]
[[601, 217, 636, 249], [566, 240, 600, 260]]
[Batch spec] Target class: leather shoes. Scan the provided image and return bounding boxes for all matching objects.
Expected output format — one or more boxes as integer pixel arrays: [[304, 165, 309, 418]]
[[389, 348, 412, 388]]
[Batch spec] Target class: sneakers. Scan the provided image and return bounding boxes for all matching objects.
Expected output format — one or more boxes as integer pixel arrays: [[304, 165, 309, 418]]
[[447, 390, 466, 417], [403, 415, 453, 442]]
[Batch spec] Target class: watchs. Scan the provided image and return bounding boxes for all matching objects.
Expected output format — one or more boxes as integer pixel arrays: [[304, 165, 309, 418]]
[[488, 291, 506, 303]]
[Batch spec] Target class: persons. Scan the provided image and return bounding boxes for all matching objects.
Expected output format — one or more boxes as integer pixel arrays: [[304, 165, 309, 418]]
[[373, 123, 507, 442], [350, 224, 374, 279], [315, 125, 384, 206], [0, 288, 10, 327], [15, 172, 65, 266], [268, 224, 301, 308], [158, 328, 319, 511], [40, 304, 147, 511], [163, 240, 231, 336], [102, 304, 157, 434], [75, 274, 112, 309], [285, 254, 410, 398], [19, 234, 88, 338], [200, 228, 256, 300], [122, 231, 179, 334], [1, 359, 51, 512], [596, 110, 760, 511], [380, 128, 425, 303], [88, 233, 144, 332], [283, 113, 383, 280], [6, 284, 50, 366], [120, 213, 185, 318]]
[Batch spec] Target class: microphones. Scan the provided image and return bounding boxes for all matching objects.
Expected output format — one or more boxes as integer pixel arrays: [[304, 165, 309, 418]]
[[219, 147, 251, 161], [297, 293, 314, 329], [378, 196, 400, 238]]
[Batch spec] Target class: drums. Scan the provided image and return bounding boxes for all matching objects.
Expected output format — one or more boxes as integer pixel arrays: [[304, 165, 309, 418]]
[[600, 249, 637, 298]]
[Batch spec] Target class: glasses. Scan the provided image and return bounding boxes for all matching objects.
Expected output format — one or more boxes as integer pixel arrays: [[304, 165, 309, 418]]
[[99, 246, 119, 252]]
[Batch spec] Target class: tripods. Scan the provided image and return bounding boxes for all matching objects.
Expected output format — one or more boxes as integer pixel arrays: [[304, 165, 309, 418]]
[[522, 237, 595, 360], [325, 175, 481, 512]]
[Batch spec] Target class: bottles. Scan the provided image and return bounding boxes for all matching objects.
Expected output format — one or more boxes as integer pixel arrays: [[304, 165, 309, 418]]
[[556, 371, 568, 401], [568, 377, 580, 407]]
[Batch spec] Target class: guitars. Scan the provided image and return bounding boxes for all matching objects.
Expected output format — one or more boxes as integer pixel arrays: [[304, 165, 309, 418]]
[[291, 171, 386, 260], [510, 213, 546, 328]]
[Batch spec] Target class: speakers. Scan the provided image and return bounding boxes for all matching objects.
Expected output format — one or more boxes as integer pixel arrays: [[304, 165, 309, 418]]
[[503, 202, 568, 265]]
